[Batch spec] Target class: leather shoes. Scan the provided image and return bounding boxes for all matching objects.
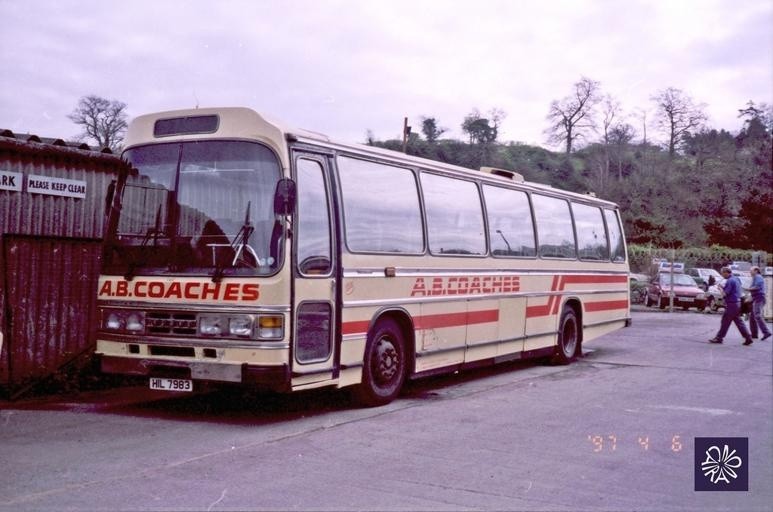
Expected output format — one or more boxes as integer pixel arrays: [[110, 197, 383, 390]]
[[709, 337, 722, 343], [743, 338, 752, 345]]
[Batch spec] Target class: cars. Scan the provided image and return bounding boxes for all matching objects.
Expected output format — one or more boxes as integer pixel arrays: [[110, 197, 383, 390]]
[[627, 271, 650, 291], [708, 276, 754, 310], [643, 273, 707, 312], [649, 254, 773, 290]]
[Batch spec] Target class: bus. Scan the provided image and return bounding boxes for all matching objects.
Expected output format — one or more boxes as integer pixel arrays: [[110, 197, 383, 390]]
[[91, 104, 633, 403]]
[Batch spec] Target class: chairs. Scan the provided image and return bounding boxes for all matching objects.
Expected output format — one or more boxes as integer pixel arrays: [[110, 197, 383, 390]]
[[195, 214, 281, 269]]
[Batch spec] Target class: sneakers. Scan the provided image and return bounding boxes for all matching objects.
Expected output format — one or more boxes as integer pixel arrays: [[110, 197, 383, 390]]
[[762, 333, 771, 340]]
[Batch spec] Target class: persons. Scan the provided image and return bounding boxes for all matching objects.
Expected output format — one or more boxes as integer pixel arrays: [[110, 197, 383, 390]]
[[708, 267, 754, 346], [742, 266, 772, 341]]
